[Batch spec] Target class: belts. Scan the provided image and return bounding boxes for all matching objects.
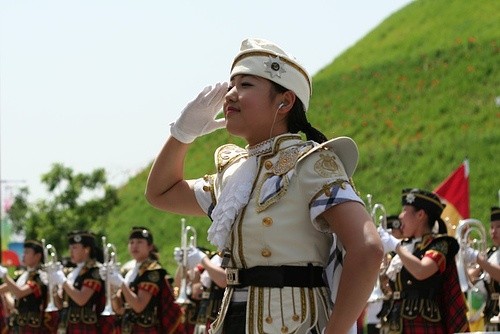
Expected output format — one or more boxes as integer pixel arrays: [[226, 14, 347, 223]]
[[224, 265, 328, 288]]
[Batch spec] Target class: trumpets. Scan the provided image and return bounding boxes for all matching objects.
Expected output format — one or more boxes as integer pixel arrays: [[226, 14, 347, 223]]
[[100, 236, 119, 317], [367, 193, 392, 303], [41, 237, 61, 313], [173, 218, 197, 304]]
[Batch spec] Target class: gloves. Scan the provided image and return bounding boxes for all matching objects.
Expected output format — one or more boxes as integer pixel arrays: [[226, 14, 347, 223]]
[[464, 245, 480, 263], [188, 245, 206, 266], [49, 262, 67, 285], [174, 247, 183, 263], [169, 81, 228, 143], [100, 264, 123, 288], [378, 227, 401, 252], [38, 269, 49, 285], [0, 265, 6, 279]]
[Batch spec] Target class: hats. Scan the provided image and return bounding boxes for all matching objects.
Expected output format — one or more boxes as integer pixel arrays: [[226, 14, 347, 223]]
[[401, 189, 446, 217], [230, 37, 313, 112], [386, 215, 400, 229], [490, 206, 500, 221], [66, 231, 97, 247], [22, 239, 44, 254], [129, 227, 152, 245]]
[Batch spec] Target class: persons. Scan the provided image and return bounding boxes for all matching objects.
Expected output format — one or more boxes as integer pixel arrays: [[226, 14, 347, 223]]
[[39, 231, 108, 334], [1, 239, 49, 334], [0, 274, 23, 334], [464, 205, 500, 331], [94, 226, 175, 334], [167, 214, 414, 334], [373, 187, 471, 334], [143, 36, 385, 334]]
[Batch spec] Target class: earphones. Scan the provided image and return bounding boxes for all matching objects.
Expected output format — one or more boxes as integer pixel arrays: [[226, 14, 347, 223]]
[[277, 102, 288, 110]]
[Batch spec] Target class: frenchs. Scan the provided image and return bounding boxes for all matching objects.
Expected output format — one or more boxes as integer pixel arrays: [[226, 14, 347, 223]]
[[455, 218, 487, 294]]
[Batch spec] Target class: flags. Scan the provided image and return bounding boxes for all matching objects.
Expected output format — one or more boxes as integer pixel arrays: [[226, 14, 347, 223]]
[[427, 159, 475, 293]]
